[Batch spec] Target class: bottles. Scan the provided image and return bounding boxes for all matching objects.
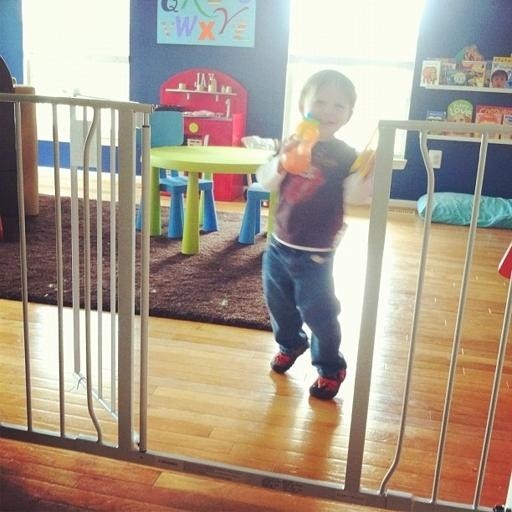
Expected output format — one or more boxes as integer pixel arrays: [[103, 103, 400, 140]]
[[279, 113, 320, 174]]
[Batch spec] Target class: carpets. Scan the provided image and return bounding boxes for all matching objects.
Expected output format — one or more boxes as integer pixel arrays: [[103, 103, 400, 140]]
[[1, 194, 350, 331]]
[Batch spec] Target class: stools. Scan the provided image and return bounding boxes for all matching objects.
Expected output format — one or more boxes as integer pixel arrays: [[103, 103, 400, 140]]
[[238, 181, 272, 244], [135, 177, 220, 236]]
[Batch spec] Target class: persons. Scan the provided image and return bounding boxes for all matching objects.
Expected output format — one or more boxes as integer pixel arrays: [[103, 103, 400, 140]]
[[253, 68, 376, 400]]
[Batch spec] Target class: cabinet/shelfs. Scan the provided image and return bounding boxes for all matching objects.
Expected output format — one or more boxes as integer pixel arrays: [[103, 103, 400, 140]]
[[424, 84, 512, 146], [156, 67, 249, 201]]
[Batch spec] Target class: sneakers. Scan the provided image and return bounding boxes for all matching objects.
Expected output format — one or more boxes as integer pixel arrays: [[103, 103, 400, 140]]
[[308, 365, 347, 401], [270, 339, 311, 375]]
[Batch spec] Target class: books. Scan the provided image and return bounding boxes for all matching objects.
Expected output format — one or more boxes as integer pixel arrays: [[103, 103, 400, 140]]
[[475, 102, 505, 139], [458, 60, 488, 87], [500, 104, 512, 140], [419, 57, 441, 88], [483, 60, 494, 87], [447, 98, 474, 138], [441, 55, 457, 86], [426, 109, 446, 136], [488, 55, 512, 89]]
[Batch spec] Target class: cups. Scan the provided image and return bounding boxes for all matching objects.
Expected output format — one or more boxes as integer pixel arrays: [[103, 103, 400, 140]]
[[221, 86, 232, 93], [179, 83, 186, 89]]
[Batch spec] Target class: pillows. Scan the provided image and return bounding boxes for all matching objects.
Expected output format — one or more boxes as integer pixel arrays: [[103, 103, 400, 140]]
[[417, 191, 512, 228]]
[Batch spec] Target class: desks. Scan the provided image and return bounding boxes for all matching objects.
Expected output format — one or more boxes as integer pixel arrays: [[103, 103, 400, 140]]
[[138, 145, 276, 256]]
